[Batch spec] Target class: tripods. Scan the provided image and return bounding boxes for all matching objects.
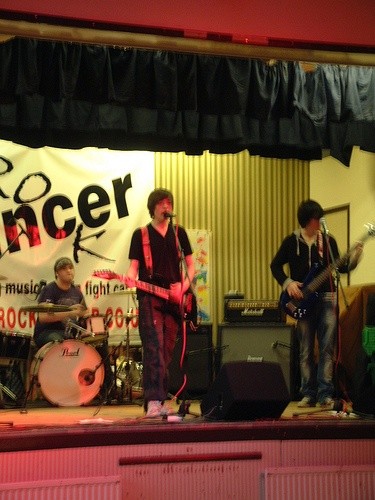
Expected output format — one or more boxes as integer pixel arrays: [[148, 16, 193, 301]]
[[292, 235, 375, 417], [136, 218, 219, 421]]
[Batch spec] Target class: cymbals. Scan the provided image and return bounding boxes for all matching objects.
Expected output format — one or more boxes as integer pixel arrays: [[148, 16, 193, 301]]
[[108, 290, 136, 295], [19, 303, 73, 312]]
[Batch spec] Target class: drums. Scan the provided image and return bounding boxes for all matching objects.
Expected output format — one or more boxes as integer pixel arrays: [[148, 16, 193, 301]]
[[0, 330, 32, 362], [85, 315, 108, 336], [30, 337, 105, 407]]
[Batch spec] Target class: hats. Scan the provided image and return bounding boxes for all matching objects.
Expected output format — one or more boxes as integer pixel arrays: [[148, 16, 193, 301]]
[[54, 257, 71, 270]]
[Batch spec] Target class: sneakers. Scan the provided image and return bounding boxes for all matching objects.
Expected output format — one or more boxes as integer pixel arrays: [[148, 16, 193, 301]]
[[144, 400, 175, 419], [321, 397, 336, 408], [297, 395, 316, 409]]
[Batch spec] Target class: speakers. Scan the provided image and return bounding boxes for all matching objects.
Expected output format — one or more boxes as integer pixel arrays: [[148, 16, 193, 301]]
[[200, 360, 291, 421]]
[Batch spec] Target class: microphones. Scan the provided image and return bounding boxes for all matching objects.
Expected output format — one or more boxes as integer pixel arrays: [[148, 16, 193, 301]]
[[318, 217, 329, 235], [14, 218, 32, 239], [161, 210, 176, 218], [35, 281, 46, 301]]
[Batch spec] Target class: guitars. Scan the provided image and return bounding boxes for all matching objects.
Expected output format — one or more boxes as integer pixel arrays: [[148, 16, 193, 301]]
[[279, 223, 375, 320], [91, 267, 193, 315]]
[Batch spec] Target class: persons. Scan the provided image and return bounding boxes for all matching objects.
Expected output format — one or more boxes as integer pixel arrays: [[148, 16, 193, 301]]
[[34, 257, 85, 345], [117, 186, 198, 422], [270, 198, 364, 408]]
